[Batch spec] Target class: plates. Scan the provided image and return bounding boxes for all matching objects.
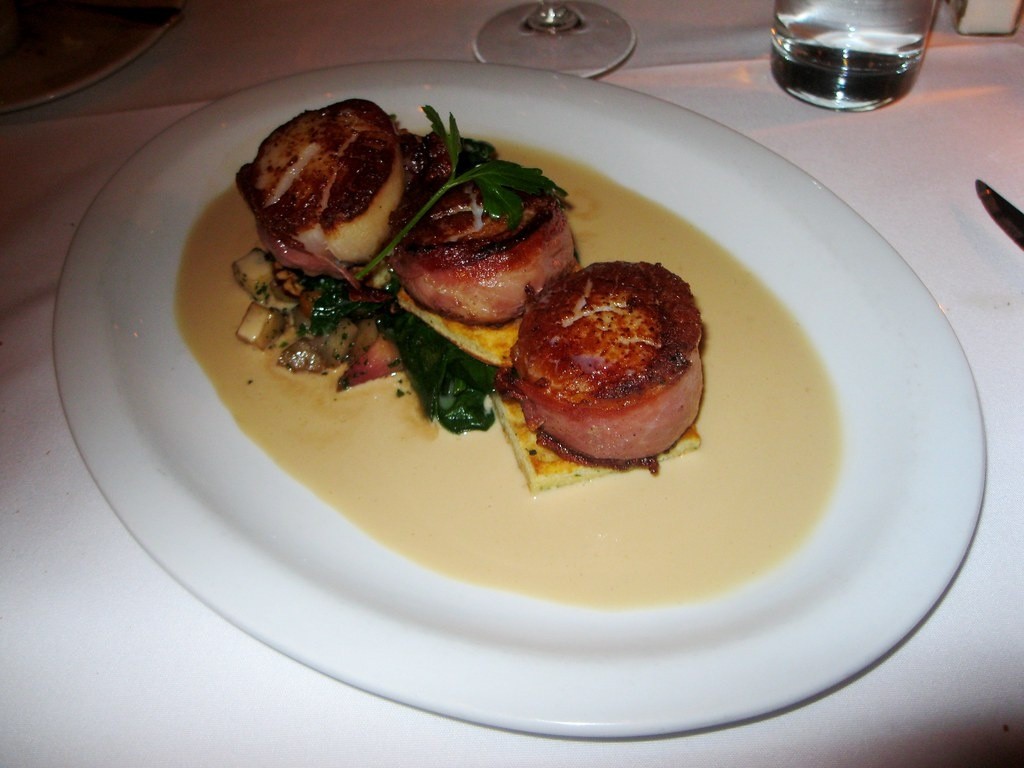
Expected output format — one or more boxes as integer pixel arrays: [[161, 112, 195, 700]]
[[54, 59, 985, 738], [2, 0, 183, 115]]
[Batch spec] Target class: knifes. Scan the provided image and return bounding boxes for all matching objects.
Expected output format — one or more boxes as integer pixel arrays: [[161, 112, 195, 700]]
[[976, 180, 1024, 250]]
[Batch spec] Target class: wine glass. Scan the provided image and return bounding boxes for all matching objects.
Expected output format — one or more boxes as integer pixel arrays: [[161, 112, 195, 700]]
[[472, 0, 636, 81]]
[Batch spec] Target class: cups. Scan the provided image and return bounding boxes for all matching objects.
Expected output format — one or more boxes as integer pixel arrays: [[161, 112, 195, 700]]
[[769, 0, 939, 112]]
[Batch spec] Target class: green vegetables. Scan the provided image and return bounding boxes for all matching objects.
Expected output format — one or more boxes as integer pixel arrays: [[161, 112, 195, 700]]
[[297, 276, 499, 434], [355, 105, 568, 282]]
